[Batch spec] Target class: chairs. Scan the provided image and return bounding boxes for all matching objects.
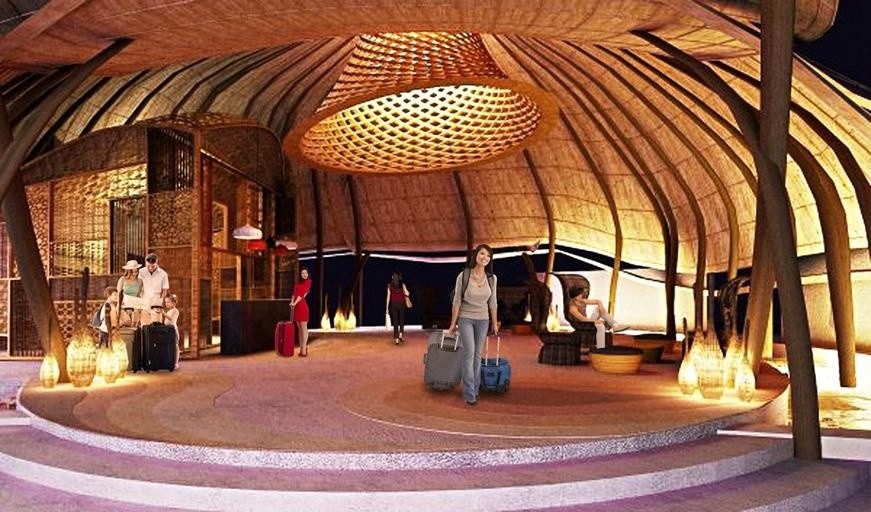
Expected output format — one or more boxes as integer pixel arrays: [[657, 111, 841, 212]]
[[526, 270, 612, 367]]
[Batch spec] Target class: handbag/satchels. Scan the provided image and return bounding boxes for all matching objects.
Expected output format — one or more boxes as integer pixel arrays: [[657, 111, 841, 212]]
[[404, 296, 412, 308]]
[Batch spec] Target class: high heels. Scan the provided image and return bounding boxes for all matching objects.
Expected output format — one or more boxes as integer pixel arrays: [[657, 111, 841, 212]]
[[298, 347, 308, 357]]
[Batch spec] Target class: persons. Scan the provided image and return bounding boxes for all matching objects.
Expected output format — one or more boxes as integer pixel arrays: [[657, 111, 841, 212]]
[[567, 285, 630, 350], [137, 253, 170, 327], [116, 259, 144, 327], [160, 292, 181, 371], [385, 270, 410, 347], [93, 286, 118, 348], [448, 243, 499, 407], [288, 265, 313, 357]]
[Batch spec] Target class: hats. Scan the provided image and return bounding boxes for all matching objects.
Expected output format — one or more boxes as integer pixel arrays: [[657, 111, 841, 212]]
[[122, 259, 142, 270]]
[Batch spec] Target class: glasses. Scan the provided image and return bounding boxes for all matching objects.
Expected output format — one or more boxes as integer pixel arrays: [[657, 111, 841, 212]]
[[147, 258, 156, 263]]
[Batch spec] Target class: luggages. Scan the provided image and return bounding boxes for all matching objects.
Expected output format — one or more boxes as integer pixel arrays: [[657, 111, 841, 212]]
[[274, 305, 295, 357], [423, 329, 462, 393], [140, 305, 176, 372], [108, 308, 143, 373], [480, 331, 511, 393]]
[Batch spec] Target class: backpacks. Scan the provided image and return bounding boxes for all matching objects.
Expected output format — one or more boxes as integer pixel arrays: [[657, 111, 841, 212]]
[[92, 303, 104, 327]]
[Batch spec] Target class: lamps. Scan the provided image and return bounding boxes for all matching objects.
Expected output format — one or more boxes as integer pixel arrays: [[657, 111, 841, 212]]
[[233, 119, 299, 256], [320, 294, 358, 334], [678, 272, 756, 404], [39, 268, 130, 388]]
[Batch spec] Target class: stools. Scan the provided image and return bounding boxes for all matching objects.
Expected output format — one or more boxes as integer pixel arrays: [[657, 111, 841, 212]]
[[591, 333, 675, 375]]
